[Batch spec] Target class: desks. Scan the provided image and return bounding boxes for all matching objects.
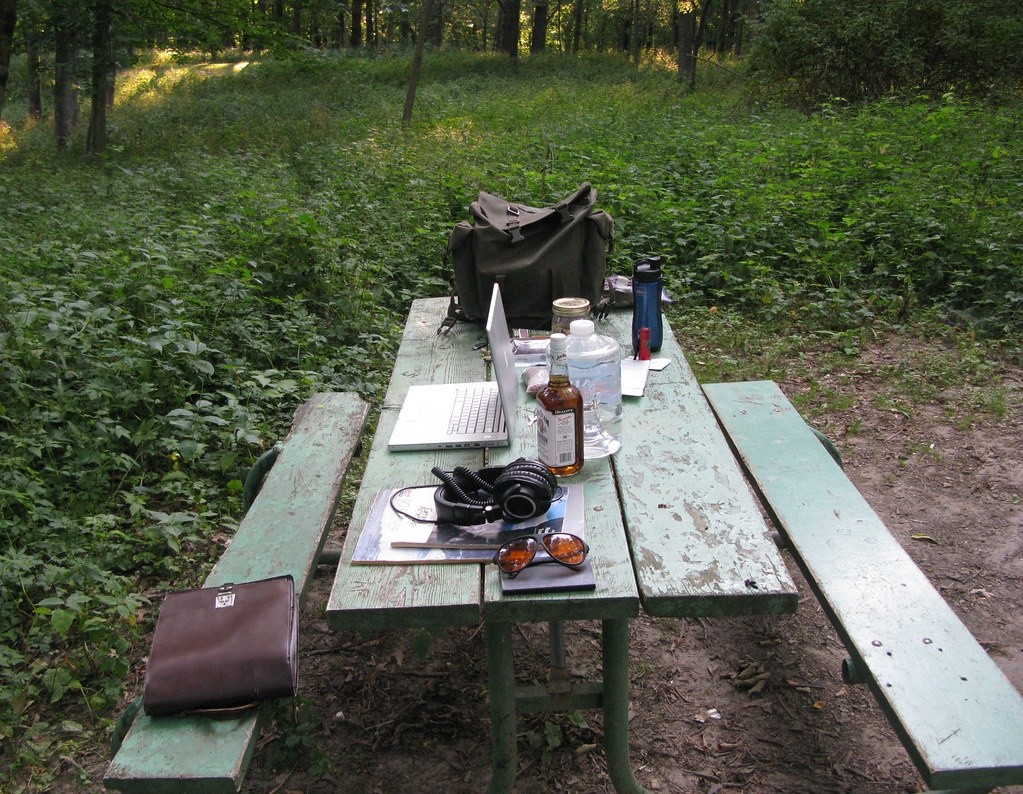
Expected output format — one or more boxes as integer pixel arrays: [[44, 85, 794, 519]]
[[324, 294, 800, 794]]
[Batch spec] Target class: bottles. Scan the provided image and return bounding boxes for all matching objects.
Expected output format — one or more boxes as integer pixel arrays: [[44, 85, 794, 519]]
[[546, 319, 622, 460], [632, 256, 664, 352], [551, 297, 590, 335], [536, 333, 585, 479]]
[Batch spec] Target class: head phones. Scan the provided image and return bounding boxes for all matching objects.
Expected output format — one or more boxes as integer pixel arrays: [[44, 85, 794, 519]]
[[431, 457, 564, 526]]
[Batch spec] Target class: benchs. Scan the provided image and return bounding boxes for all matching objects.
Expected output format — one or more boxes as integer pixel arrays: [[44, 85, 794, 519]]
[[103, 390, 371, 794], [701, 380, 1023, 794]]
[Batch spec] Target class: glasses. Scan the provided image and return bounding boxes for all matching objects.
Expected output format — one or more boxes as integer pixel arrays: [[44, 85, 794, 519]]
[[493, 532, 590, 579]]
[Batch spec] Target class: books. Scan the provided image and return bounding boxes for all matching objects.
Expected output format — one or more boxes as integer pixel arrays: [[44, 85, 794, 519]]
[[350, 484, 596, 593]]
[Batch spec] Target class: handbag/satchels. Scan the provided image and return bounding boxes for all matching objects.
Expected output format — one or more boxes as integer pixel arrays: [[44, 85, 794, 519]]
[[143, 574, 300, 715], [438, 183, 613, 339]]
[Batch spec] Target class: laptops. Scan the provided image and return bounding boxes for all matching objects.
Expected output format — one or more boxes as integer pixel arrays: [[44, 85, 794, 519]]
[[388, 283, 518, 451]]
